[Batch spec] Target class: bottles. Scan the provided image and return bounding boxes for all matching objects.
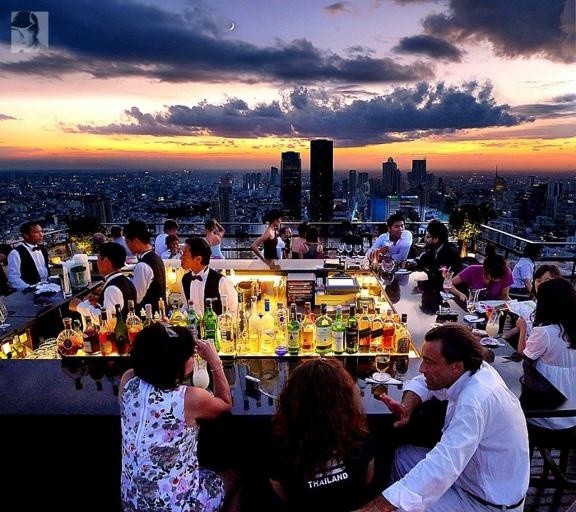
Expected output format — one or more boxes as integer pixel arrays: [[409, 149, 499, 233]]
[[60, 355, 409, 410], [57, 263, 411, 355]]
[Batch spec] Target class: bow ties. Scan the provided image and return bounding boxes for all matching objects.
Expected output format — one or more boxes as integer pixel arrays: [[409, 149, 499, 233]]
[[32, 247, 40, 252], [190, 275, 203, 281]]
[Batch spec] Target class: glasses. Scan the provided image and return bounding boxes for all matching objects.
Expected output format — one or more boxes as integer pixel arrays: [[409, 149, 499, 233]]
[[156, 320, 180, 338], [424, 235, 432, 239]]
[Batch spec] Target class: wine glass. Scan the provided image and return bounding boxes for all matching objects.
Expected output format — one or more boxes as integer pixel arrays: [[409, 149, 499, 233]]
[[442, 271, 454, 300], [486, 312, 501, 343], [0, 295, 11, 329], [345, 244, 353, 259], [354, 244, 362, 258], [337, 243, 345, 257], [467, 288, 480, 317]]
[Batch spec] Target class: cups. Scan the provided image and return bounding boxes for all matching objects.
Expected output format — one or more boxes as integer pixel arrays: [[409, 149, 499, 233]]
[[371, 248, 381, 264]]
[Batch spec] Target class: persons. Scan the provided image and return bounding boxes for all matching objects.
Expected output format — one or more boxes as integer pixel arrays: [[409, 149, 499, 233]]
[[353, 324, 531, 512], [267, 358, 375, 512], [0, 209, 576, 338], [8, 11, 47, 54], [118, 323, 243, 512], [515, 278, 576, 460]]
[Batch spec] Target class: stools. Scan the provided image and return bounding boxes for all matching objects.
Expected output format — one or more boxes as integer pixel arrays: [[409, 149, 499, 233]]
[[525, 439, 576, 512]]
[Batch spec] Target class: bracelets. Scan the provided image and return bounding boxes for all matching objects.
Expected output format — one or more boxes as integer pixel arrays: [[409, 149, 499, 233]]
[[210, 366, 223, 372]]
